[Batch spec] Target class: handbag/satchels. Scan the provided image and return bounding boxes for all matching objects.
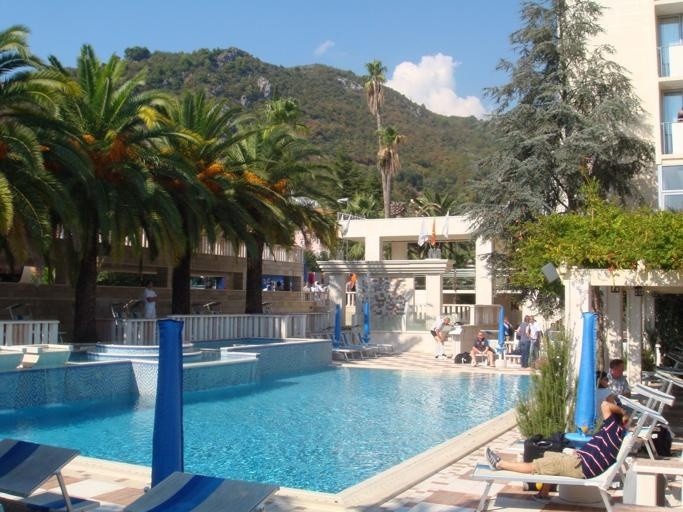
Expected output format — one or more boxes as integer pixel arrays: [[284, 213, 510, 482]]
[[454, 351, 472, 364], [523, 433, 563, 463]]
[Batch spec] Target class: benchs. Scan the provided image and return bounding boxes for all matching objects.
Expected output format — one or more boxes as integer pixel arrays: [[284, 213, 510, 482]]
[[505, 354, 522, 365], [470, 353, 499, 366]]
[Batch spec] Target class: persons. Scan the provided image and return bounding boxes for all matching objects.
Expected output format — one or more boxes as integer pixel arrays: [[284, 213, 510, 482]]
[[143, 279, 160, 318], [529, 315, 542, 362], [430, 317, 450, 358], [484, 393, 637, 480], [504, 316, 517, 341], [267, 272, 356, 305], [607, 359, 647, 406], [517, 315, 531, 368], [470, 331, 496, 367], [596, 370, 612, 418]]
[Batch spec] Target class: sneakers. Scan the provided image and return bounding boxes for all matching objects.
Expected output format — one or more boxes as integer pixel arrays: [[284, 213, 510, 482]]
[[485, 447, 503, 470], [533, 493, 552, 502]]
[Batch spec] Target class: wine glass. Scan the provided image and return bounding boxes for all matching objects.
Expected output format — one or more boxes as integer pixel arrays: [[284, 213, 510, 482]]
[[576, 424, 591, 437]]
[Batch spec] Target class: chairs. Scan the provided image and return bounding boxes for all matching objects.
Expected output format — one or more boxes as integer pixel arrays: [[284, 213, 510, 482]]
[[0, 439, 279, 512], [474, 370, 683, 512], [7, 299, 223, 342], [309, 333, 393, 362]]
